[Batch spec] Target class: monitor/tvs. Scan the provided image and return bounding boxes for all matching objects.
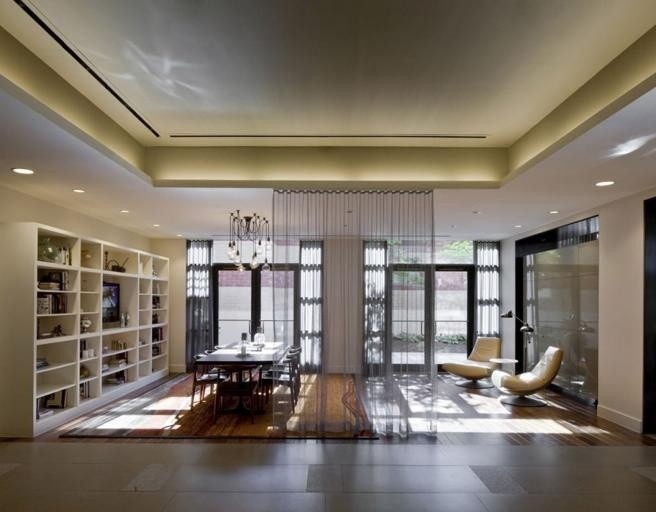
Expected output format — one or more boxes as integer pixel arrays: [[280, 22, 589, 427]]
[[103, 283, 120, 322]]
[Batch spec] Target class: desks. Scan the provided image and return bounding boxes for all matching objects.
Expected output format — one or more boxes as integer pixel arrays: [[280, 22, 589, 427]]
[[195, 341, 284, 416], [489, 358, 519, 371]]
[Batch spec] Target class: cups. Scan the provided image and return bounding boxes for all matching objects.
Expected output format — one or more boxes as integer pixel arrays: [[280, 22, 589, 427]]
[[111, 265, 120, 271], [82, 349, 94, 358]]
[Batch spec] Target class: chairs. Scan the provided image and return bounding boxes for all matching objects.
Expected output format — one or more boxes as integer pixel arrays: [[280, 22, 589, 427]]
[[442, 336, 501, 389], [191, 346, 302, 424], [491, 345, 564, 408]]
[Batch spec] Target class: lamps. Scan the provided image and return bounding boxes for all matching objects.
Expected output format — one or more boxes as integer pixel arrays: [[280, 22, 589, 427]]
[[501, 310, 535, 344], [226, 210, 270, 273]]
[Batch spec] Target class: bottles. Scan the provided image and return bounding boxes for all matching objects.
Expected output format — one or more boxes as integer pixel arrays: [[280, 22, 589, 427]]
[[253, 327, 265, 345], [120, 312, 130, 328], [240, 332, 251, 354]]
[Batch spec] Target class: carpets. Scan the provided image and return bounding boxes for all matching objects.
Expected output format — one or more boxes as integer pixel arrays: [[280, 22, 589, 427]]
[[58, 372, 380, 441]]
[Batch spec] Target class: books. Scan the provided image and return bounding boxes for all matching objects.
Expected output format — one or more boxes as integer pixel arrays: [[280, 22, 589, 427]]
[[43, 389, 67, 409], [36, 399, 40, 420], [37, 242, 73, 313], [38, 408, 54, 418], [80, 382, 91, 399]]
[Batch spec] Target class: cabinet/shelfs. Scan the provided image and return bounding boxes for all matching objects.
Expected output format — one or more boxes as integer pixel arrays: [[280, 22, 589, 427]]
[[0, 222, 170, 440]]
